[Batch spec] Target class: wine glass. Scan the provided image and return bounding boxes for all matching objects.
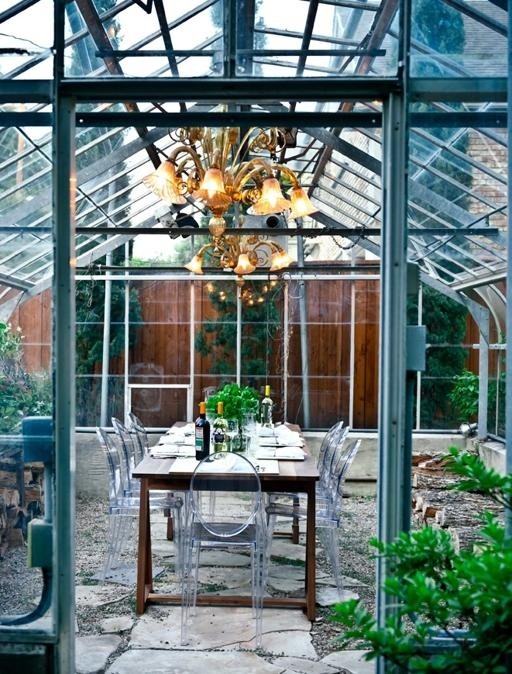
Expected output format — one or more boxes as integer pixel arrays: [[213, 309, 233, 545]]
[[226, 415, 254, 458]]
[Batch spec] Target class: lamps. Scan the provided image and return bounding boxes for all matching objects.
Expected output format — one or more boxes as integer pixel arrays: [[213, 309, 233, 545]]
[[142, 103, 321, 289]]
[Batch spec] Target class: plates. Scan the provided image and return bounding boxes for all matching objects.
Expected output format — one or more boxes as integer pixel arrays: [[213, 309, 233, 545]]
[[256, 427, 304, 461], [150, 427, 196, 457]]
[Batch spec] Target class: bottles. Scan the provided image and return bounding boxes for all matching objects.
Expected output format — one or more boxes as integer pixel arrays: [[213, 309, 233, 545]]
[[194, 401, 227, 459], [258, 385, 273, 428]]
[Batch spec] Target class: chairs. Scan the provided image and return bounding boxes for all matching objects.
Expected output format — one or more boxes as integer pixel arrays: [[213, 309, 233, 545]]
[[95, 412, 362, 650]]
